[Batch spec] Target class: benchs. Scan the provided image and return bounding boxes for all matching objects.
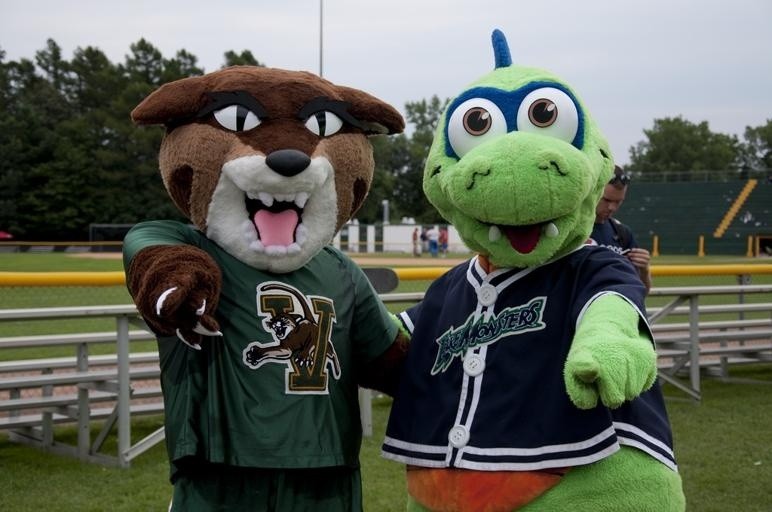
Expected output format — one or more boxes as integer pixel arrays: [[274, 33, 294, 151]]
[[614, 179, 772, 255], [0, 282, 769, 437]]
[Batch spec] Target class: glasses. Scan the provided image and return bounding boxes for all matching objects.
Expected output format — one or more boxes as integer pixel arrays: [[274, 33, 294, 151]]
[[610, 173, 630, 185]]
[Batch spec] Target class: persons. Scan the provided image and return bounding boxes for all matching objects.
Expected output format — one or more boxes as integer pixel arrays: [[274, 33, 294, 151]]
[[413, 226, 448, 258], [584, 163, 651, 297]]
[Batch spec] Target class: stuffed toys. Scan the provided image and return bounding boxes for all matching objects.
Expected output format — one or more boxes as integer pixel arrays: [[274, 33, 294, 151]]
[[385, 28, 686, 512], [123, 63, 411, 512]]
